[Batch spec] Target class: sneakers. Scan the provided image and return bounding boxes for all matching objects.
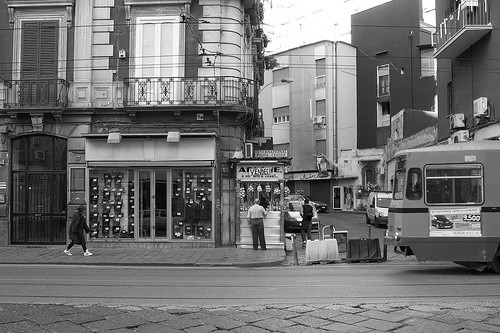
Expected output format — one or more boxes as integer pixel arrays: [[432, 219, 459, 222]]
[[84, 249, 93, 256], [64, 249, 72, 255]]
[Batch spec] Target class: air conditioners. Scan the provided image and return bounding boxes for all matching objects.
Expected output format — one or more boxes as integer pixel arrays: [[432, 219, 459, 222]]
[[313, 116, 322, 124], [473, 96, 487, 118], [245, 143, 253, 159], [450, 113, 465, 130], [453, 130, 470, 144]]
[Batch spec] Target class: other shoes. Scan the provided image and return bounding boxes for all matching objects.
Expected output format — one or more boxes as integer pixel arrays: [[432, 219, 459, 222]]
[[303, 240, 307, 243]]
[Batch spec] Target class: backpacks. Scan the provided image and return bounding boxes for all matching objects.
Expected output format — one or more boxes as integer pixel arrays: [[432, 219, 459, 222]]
[[304, 206, 314, 221]]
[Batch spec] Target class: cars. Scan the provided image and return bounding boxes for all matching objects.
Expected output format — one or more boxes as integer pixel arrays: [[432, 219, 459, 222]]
[[141, 208, 184, 230], [283, 193, 328, 232]]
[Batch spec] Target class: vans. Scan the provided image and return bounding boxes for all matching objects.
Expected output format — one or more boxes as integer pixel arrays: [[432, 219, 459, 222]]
[[364, 190, 393, 229]]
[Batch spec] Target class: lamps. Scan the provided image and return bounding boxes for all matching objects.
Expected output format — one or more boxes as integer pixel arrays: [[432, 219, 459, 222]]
[[107, 132, 122, 144], [166, 132, 181, 143]]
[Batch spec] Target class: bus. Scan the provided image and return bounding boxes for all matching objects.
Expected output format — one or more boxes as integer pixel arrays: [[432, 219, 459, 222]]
[[383, 138, 500, 275]]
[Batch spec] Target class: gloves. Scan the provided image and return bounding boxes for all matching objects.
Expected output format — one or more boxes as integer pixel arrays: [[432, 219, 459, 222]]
[[89, 229, 94, 233], [74, 232, 81, 239]]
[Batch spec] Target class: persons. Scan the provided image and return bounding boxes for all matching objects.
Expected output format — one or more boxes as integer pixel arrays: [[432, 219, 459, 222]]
[[63, 206, 94, 256], [247, 198, 267, 250], [289, 203, 294, 211], [259, 194, 270, 211], [300, 199, 314, 244]]
